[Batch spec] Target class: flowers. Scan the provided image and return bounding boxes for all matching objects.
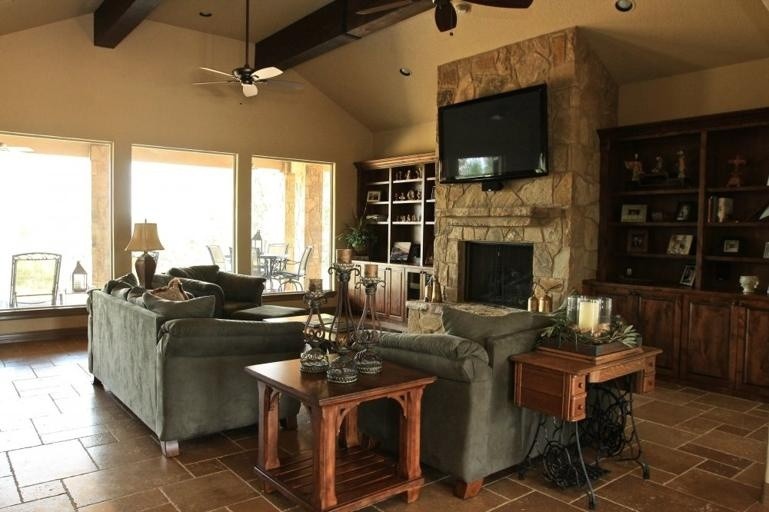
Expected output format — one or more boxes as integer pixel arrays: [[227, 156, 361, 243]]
[[353, 1, 536, 34]]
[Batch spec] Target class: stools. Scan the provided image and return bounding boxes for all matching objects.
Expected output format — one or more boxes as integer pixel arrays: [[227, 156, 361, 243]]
[[234, 303, 306, 320]]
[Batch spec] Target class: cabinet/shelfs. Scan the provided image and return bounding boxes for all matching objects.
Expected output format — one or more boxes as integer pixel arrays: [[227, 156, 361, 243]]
[[597, 107, 769, 296], [404, 267, 437, 323], [351, 257, 406, 323], [589, 285, 680, 378], [355, 150, 438, 266], [680, 285, 767, 398]]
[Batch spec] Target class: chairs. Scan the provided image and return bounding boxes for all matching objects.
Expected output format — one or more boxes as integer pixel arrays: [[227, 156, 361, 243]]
[[9, 251, 64, 309], [207, 232, 312, 294]]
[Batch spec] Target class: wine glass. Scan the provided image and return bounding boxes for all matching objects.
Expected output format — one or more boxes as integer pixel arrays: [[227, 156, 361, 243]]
[[739, 276, 759, 293]]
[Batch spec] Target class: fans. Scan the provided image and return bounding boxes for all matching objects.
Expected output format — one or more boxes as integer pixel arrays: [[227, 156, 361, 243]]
[[191, 2, 287, 97]]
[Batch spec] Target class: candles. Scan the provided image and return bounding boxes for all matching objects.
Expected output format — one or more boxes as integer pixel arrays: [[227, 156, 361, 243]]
[[577, 301, 600, 331], [310, 277, 323, 291], [336, 247, 353, 265], [364, 264, 378, 279]]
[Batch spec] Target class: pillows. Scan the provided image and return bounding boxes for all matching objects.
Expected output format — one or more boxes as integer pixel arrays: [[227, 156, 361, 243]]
[[105, 264, 222, 320], [440, 303, 536, 341]]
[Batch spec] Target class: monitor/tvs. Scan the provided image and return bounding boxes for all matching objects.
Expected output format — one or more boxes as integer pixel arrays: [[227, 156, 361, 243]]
[[438, 83, 549, 183]]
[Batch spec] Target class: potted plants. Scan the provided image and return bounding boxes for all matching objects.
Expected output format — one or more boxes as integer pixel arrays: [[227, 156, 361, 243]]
[[335, 201, 373, 257]]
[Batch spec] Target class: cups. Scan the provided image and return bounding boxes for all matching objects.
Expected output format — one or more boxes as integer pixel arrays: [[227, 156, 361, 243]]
[[566, 294, 612, 335]]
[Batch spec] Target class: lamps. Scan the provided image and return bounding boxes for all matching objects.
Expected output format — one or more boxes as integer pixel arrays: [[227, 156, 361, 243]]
[[71, 260, 89, 292], [123, 217, 165, 290]]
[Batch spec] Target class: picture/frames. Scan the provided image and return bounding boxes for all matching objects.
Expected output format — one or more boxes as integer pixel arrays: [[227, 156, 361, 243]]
[[366, 189, 380, 201], [626, 228, 649, 253], [678, 263, 696, 286], [619, 202, 648, 222]]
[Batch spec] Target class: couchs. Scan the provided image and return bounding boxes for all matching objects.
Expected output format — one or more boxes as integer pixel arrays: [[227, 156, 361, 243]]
[[351, 302, 597, 497], [87, 264, 307, 463]]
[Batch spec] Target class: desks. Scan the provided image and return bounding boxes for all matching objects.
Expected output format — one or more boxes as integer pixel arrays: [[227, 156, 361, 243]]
[[506, 347, 663, 511], [241, 352, 437, 512]]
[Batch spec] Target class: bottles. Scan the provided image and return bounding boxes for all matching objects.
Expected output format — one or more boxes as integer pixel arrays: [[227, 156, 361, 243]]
[[425, 274, 441, 303], [707, 195, 719, 223], [540, 293, 553, 312], [527, 293, 537, 310]]
